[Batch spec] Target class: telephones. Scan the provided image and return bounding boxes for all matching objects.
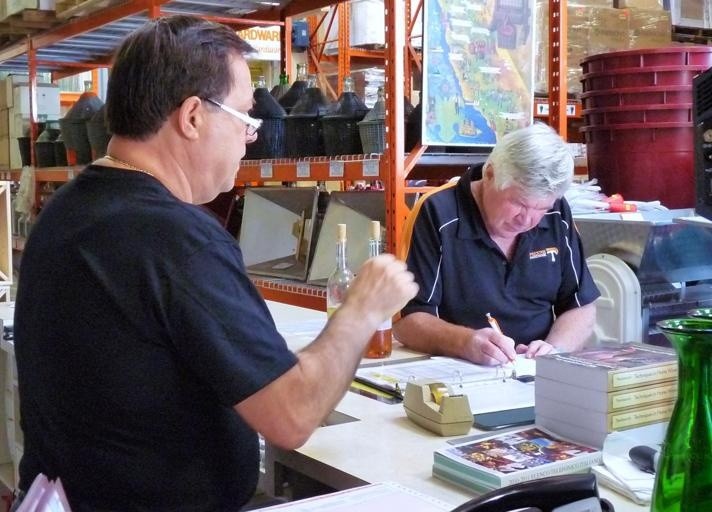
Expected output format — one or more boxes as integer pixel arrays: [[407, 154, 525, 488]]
[[450, 474, 615, 512]]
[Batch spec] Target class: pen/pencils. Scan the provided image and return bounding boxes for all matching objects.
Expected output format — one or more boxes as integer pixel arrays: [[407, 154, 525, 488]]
[[486, 313, 517, 366]]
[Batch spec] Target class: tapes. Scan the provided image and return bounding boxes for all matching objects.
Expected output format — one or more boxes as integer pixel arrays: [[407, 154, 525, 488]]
[[429, 382, 450, 405]]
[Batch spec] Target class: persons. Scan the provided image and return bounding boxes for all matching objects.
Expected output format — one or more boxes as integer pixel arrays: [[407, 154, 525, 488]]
[[11, 11, 421, 512], [390, 120, 603, 369]]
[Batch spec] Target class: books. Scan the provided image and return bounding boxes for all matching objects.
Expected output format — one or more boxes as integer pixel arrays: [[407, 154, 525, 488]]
[[354, 355, 536, 428], [349, 380, 400, 404], [432, 425, 603, 496], [592, 419, 676, 505], [535, 342, 677, 450], [431, 353, 535, 380]]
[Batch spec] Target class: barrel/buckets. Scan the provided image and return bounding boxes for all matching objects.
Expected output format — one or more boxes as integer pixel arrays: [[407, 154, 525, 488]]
[[579, 46, 712, 209]]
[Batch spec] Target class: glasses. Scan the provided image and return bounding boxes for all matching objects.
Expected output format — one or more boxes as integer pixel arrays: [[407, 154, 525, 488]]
[[177, 94, 263, 136]]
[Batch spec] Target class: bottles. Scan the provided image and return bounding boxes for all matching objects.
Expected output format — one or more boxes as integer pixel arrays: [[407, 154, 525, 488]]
[[326, 222, 392, 360], [649, 307, 712, 512], [17, 213, 33, 239], [36, 79, 106, 165], [250, 63, 386, 159]]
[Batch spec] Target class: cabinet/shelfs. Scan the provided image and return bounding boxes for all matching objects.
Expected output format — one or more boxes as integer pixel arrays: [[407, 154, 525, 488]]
[[1, 2, 568, 321]]
